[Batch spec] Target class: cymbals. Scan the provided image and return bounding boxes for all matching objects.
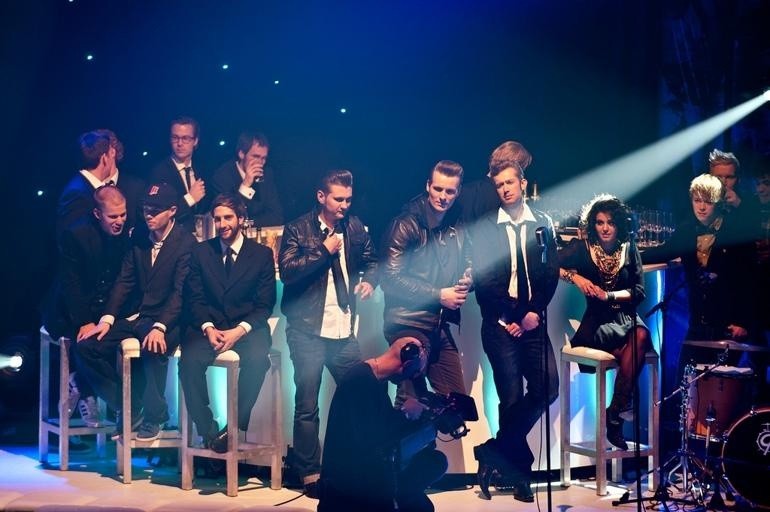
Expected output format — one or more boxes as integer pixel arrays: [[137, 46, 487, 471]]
[[682, 339, 758, 352]]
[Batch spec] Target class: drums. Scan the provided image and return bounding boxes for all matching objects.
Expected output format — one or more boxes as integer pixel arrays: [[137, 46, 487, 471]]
[[721, 408, 769, 508], [680, 364, 755, 442]]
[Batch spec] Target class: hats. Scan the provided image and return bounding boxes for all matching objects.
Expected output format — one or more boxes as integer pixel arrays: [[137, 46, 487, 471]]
[[135, 180, 179, 209]]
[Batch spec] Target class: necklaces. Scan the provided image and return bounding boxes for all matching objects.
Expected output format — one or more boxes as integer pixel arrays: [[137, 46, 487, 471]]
[[374, 357, 379, 376]]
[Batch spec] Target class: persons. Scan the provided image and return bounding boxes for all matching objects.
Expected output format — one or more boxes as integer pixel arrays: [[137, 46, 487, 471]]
[[640, 149, 770, 416], [316, 337, 448, 512], [461, 141, 559, 503], [381, 158, 475, 410], [558, 194, 652, 451], [39, 114, 276, 453], [277, 168, 380, 500]]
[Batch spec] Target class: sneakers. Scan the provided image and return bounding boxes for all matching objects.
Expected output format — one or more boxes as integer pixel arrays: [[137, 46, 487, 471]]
[[77, 394, 102, 428], [134, 404, 167, 441], [57, 370, 82, 418], [109, 403, 145, 441]]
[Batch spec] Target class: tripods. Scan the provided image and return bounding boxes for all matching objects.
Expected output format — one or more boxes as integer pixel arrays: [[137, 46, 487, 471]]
[[618, 406, 733, 511]]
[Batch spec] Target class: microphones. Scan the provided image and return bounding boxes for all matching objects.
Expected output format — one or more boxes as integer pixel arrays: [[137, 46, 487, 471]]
[[628, 232, 638, 255], [536, 228, 550, 258]]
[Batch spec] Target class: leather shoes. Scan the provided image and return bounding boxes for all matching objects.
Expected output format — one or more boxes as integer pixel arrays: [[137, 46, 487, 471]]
[[201, 420, 219, 448], [605, 390, 635, 450], [298, 473, 321, 499], [512, 479, 535, 503], [210, 424, 228, 453], [473, 443, 495, 500]]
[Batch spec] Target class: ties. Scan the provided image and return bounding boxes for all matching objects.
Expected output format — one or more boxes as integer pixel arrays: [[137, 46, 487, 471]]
[[331, 252, 350, 311], [509, 222, 530, 314], [184, 167, 191, 194], [224, 247, 233, 280]]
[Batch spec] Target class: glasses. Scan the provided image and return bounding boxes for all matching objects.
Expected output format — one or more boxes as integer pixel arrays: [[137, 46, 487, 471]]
[[169, 133, 196, 144]]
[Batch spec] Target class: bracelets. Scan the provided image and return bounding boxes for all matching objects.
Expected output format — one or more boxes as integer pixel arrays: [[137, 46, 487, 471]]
[[400, 406, 409, 419], [563, 271, 574, 284], [607, 291, 615, 302]]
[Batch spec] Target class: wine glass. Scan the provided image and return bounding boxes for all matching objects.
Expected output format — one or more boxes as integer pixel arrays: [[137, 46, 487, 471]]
[[541, 195, 677, 248]]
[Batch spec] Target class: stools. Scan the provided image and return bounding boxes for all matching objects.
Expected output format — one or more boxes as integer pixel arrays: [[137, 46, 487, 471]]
[[113, 331, 196, 486], [558, 339, 662, 499], [32, 323, 125, 478], [180, 340, 285, 501]]
